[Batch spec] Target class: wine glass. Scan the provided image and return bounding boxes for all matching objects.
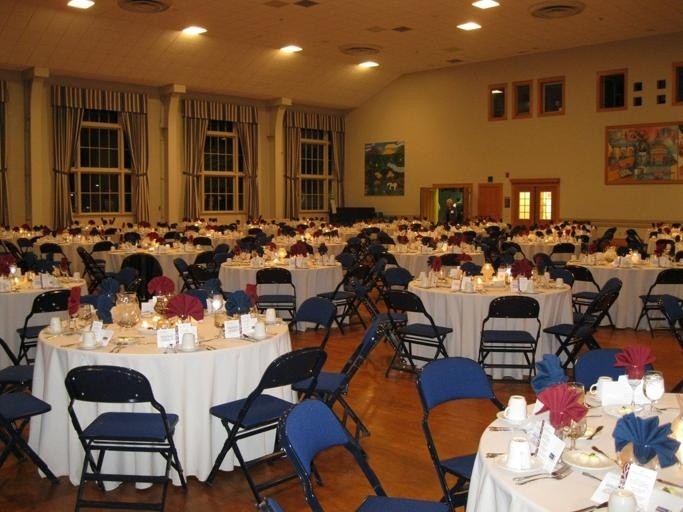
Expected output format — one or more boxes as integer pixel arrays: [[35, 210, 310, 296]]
[[565, 370, 666, 448]]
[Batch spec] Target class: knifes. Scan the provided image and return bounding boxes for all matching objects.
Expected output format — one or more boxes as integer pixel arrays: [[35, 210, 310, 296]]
[[656, 478, 682, 487]]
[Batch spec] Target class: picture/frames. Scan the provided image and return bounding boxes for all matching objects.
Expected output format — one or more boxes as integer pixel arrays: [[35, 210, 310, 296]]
[[604, 121, 682, 186]]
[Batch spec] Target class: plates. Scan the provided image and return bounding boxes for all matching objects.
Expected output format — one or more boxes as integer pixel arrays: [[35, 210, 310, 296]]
[[493, 454, 542, 472], [562, 448, 615, 471], [496, 411, 533, 425]]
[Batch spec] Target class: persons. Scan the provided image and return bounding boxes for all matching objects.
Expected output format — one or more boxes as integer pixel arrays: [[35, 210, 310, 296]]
[[437, 197, 457, 224]]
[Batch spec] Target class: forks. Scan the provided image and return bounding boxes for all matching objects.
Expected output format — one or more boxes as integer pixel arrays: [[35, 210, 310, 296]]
[[512, 463, 571, 485]]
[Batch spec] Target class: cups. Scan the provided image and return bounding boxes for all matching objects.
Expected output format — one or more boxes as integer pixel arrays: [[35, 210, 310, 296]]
[[506, 436, 531, 468], [503, 394, 527, 420], [419, 264, 563, 292], [45, 292, 278, 352], [609, 489, 637, 511]]
